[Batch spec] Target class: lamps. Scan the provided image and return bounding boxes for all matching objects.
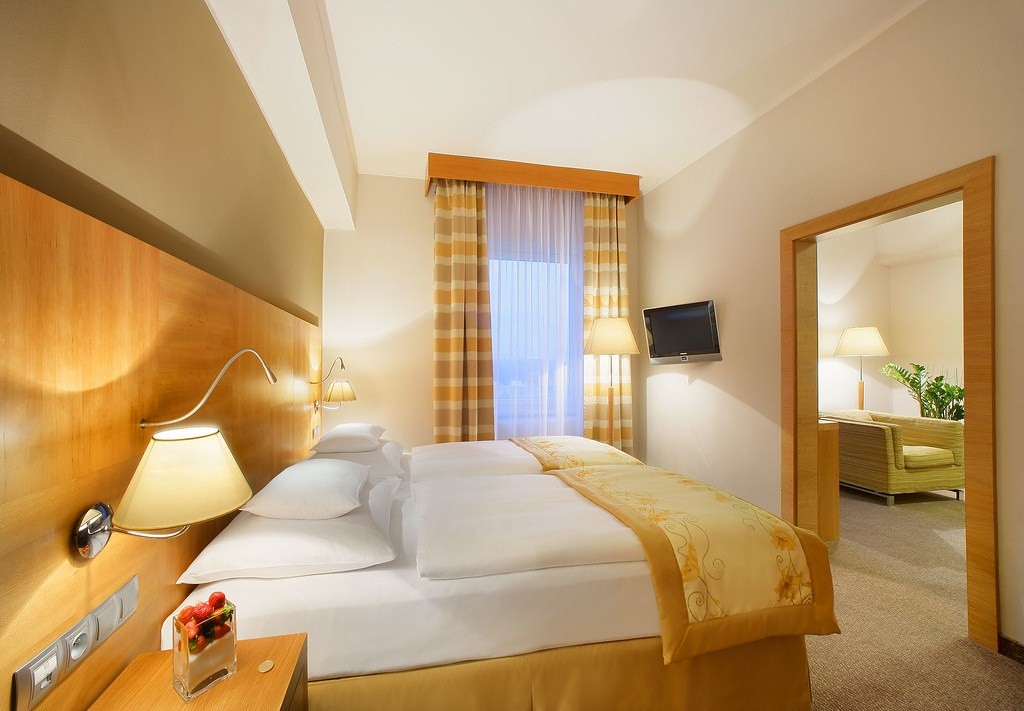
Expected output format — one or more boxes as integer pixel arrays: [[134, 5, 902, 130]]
[[584, 318, 640, 436], [315, 380, 356, 410], [141, 348, 277, 428], [833, 327, 890, 410], [74, 425, 253, 559], [311, 357, 346, 384]]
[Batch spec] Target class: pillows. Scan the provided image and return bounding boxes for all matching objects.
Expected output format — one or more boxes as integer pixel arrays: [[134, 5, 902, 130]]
[[309, 423, 386, 453], [304, 439, 405, 474], [174, 473, 401, 584], [239, 459, 371, 520]]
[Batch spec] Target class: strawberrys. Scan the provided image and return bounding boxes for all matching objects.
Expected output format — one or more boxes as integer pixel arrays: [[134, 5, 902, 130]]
[[175, 591, 233, 655]]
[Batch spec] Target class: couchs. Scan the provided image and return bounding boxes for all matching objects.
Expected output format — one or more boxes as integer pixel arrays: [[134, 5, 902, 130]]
[[818, 410, 966, 506]]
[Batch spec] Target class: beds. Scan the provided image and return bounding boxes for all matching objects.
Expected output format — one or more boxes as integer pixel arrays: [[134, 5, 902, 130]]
[[161, 436, 841, 711]]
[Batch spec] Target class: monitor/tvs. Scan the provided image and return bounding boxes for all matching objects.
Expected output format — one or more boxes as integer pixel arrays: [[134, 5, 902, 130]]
[[642, 299, 721, 359]]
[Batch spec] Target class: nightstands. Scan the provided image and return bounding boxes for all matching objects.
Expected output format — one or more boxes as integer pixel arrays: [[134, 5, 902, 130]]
[[86, 633, 308, 711]]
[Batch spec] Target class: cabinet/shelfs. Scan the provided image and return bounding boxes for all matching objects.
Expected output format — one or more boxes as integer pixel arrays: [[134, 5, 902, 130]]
[[818, 420, 840, 541]]
